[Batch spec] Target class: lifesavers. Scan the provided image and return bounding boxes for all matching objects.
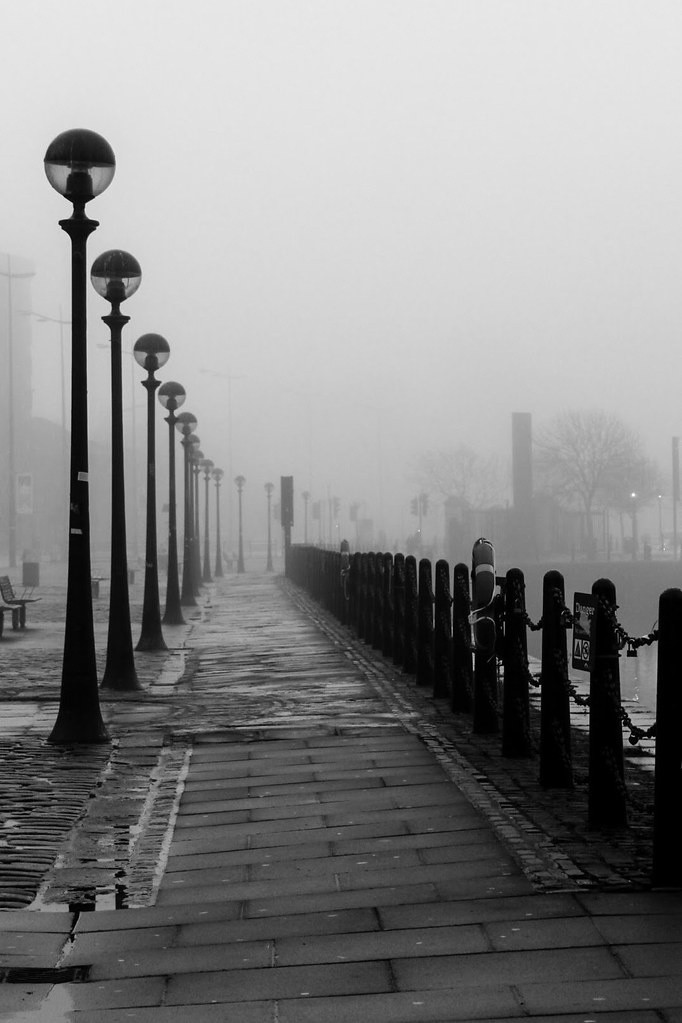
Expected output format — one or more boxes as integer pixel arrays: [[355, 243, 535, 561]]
[[470, 535, 496, 623], [339, 539, 351, 592]]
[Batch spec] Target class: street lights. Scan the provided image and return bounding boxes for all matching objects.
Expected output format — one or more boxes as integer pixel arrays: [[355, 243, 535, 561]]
[[211, 468, 225, 580], [38, 129, 114, 748], [190, 448, 203, 599], [199, 459, 218, 582], [297, 489, 312, 544], [264, 482, 279, 573], [86, 248, 147, 692], [130, 333, 172, 656], [153, 381, 189, 627], [181, 432, 199, 603], [232, 475, 247, 571], [175, 411, 196, 606]]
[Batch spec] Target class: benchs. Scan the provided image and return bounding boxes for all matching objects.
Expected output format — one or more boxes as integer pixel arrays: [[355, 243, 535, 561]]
[[0, 597, 23, 639], [0, 576, 41, 627]]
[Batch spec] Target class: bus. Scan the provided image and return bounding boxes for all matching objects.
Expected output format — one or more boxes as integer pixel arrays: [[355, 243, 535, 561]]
[[622, 537, 641, 554]]
